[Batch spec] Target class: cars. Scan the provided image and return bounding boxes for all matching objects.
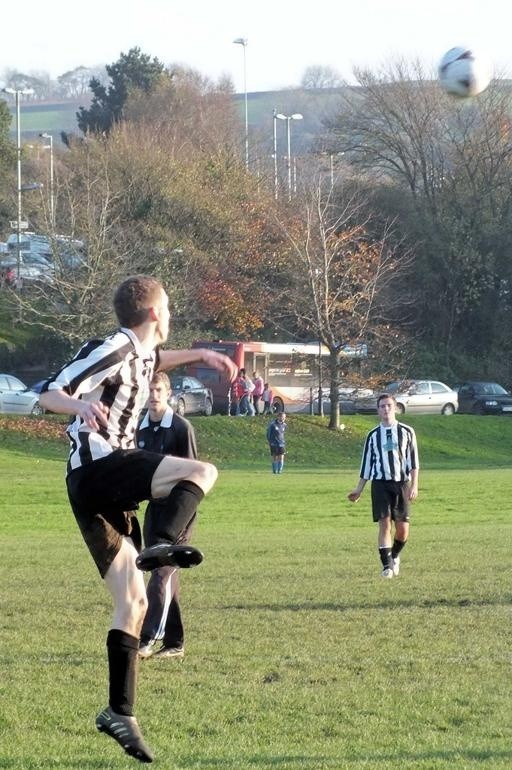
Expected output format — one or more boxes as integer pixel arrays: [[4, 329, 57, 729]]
[[453, 382, 512, 415], [0, 375, 48, 415], [3, 263, 55, 295], [167, 375, 213, 416], [353, 380, 458, 415]]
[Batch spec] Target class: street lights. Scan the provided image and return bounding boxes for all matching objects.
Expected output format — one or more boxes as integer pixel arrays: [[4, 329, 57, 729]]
[[277, 112, 303, 199], [236, 32, 253, 178], [38, 131, 67, 242], [0, 87, 37, 284]]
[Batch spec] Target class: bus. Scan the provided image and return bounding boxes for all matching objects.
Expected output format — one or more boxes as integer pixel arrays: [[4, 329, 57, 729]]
[[187, 339, 374, 414]]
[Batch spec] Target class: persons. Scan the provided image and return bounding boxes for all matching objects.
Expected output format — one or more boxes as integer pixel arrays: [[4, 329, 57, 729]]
[[38, 273, 241, 765], [4, 266, 16, 289], [239, 370, 249, 416], [259, 383, 274, 416], [347, 393, 420, 580], [133, 370, 198, 662], [251, 372, 265, 416], [230, 375, 248, 416], [267, 412, 289, 475], [240, 368, 257, 417]]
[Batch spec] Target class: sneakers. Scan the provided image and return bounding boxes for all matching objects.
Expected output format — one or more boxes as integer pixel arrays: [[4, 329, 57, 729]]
[[136, 543, 204, 572], [393, 556, 400, 576], [382, 569, 393, 578], [96, 707, 154, 763], [137, 639, 156, 658], [150, 647, 184, 659]]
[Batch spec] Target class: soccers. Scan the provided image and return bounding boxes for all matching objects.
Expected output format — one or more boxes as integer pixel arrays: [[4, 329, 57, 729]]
[[439, 46, 492, 94]]
